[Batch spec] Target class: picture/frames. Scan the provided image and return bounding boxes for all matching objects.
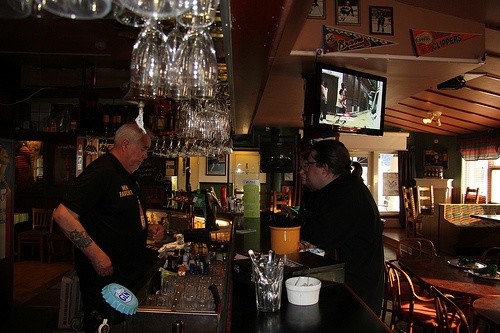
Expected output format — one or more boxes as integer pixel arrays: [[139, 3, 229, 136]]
[[368, 5, 394, 36], [306, 0, 327, 20], [205, 154, 227, 176], [334, 0, 361, 27]]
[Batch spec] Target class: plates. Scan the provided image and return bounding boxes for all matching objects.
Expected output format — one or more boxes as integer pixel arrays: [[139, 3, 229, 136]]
[[447, 259, 487, 270], [468, 269, 500, 280]]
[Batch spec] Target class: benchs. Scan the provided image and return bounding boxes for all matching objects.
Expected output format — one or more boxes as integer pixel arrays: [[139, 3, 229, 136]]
[[420, 203, 500, 255]]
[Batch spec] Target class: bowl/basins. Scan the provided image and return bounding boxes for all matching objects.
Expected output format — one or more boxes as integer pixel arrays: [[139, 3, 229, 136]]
[[286, 302, 321, 328], [285, 276, 322, 306]]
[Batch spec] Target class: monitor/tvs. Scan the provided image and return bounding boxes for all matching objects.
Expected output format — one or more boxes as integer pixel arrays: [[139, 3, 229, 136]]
[[310, 62, 386, 137]]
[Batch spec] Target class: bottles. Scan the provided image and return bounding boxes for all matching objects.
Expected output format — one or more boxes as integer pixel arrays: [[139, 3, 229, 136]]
[[424, 168, 439, 177], [112, 107, 121, 135], [156, 106, 166, 130], [103, 106, 110, 134], [70, 108, 77, 132], [167, 242, 217, 275], [149, 107, 156, 131]]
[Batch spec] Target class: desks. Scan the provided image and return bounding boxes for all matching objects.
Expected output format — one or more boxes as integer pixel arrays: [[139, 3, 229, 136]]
[[14, 211, 29, 260], [469, 214, 500, 256], [412, 254, 500, 333]]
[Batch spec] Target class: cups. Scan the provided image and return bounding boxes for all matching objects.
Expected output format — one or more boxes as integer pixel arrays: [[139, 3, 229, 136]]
[[251, 255, 284, 313], [256, 311, 281, 333], [269, 224, 301, 255]]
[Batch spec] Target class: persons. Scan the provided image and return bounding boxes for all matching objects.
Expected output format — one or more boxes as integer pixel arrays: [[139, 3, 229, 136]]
[[336, 83, 346, 124], [320, 80, 328, 120], [53, 125, 151, 333], [294, 139, 384, 318]]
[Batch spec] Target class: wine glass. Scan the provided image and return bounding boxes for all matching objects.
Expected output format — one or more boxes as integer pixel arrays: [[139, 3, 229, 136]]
[[0, 0, 233, 158]]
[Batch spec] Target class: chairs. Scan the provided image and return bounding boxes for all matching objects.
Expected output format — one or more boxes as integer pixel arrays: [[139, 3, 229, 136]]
[[383, 261, 455, 333], [395, 237, 438, 266], [416, 185, 435, 213], [429, 284, 473, 333], [400, 185, 423, 238], [47, 229, 70, 265], [464, 186, 480, 204], [17, 207, 56, 263]]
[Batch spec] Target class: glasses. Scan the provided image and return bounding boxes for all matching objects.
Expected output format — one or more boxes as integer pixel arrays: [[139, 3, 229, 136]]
[[302, 160, 320, 168]]
